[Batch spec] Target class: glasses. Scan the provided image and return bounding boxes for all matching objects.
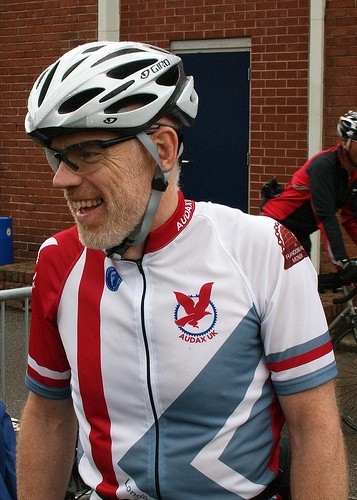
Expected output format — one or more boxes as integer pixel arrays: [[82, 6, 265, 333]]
[[43, 130, 160, 174]]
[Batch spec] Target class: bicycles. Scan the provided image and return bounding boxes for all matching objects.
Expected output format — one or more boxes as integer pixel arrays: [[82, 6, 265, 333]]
[[317, 258, 357, 431]]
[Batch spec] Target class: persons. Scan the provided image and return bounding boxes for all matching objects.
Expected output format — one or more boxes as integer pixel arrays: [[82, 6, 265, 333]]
[[260, 110, 357, 284], [16, 40, 349, 499]]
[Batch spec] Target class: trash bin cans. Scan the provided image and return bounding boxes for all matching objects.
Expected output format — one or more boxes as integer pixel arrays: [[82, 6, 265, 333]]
[[0, 217, 13, 266]]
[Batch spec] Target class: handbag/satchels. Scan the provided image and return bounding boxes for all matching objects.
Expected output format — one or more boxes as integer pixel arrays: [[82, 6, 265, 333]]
[[261, 178, 283, 199]]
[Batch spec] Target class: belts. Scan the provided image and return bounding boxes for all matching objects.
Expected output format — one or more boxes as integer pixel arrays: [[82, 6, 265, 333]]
[[249, 476, 283, 500]]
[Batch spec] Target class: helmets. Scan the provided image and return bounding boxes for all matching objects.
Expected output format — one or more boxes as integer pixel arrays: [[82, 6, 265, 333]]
[[337, 110, 357, 141], [24, 40, 199, 145]]
